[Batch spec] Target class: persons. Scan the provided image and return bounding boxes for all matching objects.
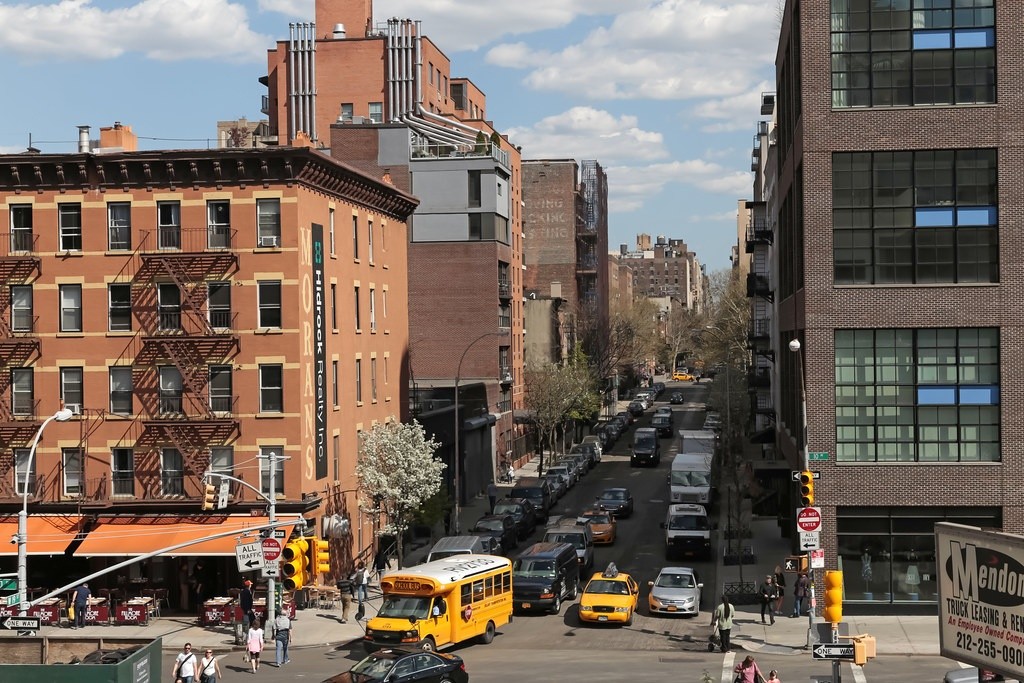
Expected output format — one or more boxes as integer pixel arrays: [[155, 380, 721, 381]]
[[341, 546, 391, 623], [648, 370, 665, 386], [734, 655, 780, 683], [196, 649, 221, 683], [486, 478, 498, 513], [861, 548, 936, 584], [696, 375, 700, 384], [506, 465, 515, 484], [177, 563, 206, 613], [759, 561, 810, 626], [172, 643, 198, 683], [271, 610, 293, 667], [711, 595, 734, 654], [441, 503, 452, 535], [241, 580, 254, 634], [246, 620, 264, 673], [70, 584, 92, 630]]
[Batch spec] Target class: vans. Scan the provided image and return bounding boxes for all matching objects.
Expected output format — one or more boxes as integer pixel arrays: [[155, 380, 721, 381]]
[[510, 542, 581, 614], [427, 536, 483, 566]]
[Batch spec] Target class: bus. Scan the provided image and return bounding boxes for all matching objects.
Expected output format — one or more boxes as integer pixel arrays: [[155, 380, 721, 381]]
[[363, 553, 514, 656]]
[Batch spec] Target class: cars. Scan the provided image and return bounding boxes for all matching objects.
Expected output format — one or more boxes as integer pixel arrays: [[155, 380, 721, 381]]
[[649, 566, 704, 617], [467, 367, 725, 567], [577, 562, 640, 627], [319, 646, 469, 683]]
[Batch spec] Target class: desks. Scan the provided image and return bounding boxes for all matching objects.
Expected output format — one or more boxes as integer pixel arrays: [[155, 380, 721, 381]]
[[203, 596, 234, 606], [303, 585, 337, 590], [86, 598, 108, 607], [37, 598, 61, 622], [126, 598, 154, 621]]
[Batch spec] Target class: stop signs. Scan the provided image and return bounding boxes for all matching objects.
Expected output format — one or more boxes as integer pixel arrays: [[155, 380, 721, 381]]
[[260, 538, 281, 559], [797, 508, 822, 532]]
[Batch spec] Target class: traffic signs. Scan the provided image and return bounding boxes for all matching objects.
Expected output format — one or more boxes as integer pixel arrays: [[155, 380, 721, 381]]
[[235, 541, 265, 572], [791, 470, 821, 483], [220, 480, 229, 509], [812, 643, 854, 660], [261, 559, 280, 577], [258, 529, 286, 539], [3, 616, 41, 631], [800, 531, 819, 551]]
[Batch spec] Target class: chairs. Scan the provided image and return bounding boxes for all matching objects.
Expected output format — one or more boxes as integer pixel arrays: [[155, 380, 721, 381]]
[[226, 588, 267, 599], [307, 590, 341, 610], [26, 586, 170, 622]]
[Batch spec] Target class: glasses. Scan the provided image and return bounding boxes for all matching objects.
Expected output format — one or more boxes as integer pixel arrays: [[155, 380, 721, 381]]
[[206, 652, 212, 653], [185, 648, 190, 650]]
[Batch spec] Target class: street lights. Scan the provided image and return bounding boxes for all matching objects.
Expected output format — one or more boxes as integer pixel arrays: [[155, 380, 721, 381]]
[[707, 326, 732, 463], [453, 330, 513, 533], [789, 338, 818, 649], [11, 408, 74, 633]]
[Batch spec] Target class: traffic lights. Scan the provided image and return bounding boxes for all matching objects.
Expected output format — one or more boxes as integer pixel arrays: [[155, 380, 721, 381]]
[[784, 558, 805, 574], [311, 539, 330, 576], [281, 542, 306, 592], [202, 484, 216, 511], [852, 641, 866, 665], [799, 471, 815, 507]]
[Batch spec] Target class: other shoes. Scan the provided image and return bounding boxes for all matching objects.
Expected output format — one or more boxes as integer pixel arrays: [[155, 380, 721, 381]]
[[276, 663, 280, 667], [284, 659, 290, 664], [340, 619, 346, 623], [762, 619, 766, 623], [775, 611, 780, 614], [793, 613, 800, 617], [771, 620, 775, 625], [779, 611, 783, 614]]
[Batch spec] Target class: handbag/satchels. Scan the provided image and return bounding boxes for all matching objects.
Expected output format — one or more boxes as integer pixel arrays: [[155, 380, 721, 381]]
[[754, 671, 762, 683], [199, 673, 208, 682], [68, 606, 75, 620], [177, 668, 180, 677], [805, 587, 809, 596], [243, 650, 251, 663], [734, 678, 744, 683]]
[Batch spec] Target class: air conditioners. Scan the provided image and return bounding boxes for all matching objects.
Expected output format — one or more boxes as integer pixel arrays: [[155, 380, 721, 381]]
[[262, 236, 277, 246], [64, 404, 83, 415]]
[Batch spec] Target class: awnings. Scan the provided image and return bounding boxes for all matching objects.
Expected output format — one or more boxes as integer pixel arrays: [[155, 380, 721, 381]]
[[0, 516, 299, 558]]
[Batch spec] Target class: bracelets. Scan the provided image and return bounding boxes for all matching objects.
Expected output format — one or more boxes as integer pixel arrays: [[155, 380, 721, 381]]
[[72, 601, 74, 603]]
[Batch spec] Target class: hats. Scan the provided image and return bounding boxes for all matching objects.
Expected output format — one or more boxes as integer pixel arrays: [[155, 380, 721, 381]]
[[245, 580, 253, 586]]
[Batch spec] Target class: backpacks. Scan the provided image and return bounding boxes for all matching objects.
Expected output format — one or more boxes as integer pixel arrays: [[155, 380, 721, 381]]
[[356, 568, 366, 584]]
